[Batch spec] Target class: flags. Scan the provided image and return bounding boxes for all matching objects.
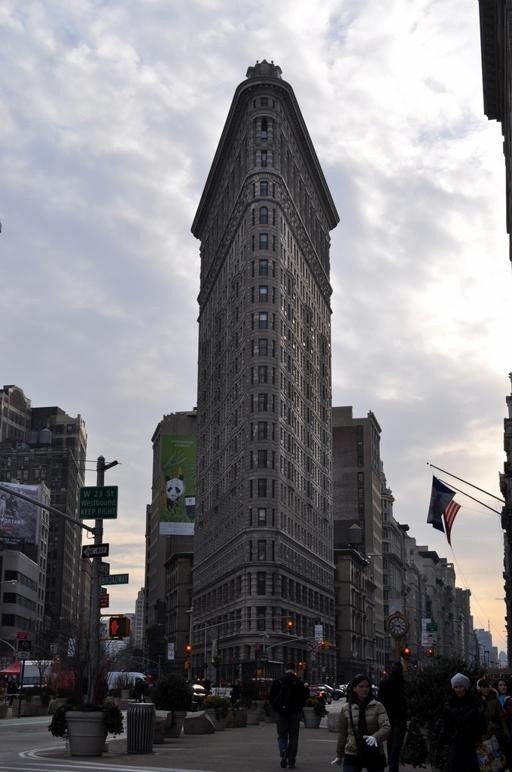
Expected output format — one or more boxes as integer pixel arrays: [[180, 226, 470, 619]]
[[442, 498, 460, 546], [426, 475, 456, 533]]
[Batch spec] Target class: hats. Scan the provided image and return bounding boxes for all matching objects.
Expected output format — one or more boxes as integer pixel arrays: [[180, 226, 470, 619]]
[[451, 672, 471, 690]]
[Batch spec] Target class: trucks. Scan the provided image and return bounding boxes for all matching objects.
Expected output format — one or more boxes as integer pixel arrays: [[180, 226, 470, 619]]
[[17, 660, 54, 694]]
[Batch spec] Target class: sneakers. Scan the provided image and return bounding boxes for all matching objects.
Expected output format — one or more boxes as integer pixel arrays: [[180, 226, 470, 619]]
[[279, 756, 297, 768]]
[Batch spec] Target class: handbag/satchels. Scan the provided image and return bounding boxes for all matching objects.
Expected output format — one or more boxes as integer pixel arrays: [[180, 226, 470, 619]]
[[359, 748, 388, 769]]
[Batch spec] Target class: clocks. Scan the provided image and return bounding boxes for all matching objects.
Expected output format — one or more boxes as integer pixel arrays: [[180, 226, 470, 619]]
[[387, 611, 408, 638]]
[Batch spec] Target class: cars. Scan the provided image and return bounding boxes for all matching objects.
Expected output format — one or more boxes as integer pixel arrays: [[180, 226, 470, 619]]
[[308, 684, 345, 700], [192, 684, 207, 702]]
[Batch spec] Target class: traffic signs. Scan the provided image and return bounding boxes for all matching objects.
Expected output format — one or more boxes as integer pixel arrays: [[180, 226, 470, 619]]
[[78, 486, 117, 518], [427, 622, 438, 632], [82, 543, 108, 559], [100, 574, 128, 584], [96, 561, 109, 575]]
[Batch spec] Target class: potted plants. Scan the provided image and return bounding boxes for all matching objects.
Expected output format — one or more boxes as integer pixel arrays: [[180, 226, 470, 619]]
[[302, 698, 330, 728], [50, 693, 124, 756], [149, 670, 262, 738]]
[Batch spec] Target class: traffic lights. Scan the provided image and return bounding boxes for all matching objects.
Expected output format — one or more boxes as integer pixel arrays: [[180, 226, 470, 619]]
[[185, 645, 192, 653], [288, 620, 294, 631], [403, 647, 411, 657], [109, 618, 130, 638], [297, 660, 304, 669]]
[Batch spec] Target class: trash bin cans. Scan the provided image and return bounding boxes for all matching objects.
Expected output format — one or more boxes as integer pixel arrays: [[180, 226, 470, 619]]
[[234, 708, 247, 725], [127, 704, 155, 752], [31, 697, 43, 717]]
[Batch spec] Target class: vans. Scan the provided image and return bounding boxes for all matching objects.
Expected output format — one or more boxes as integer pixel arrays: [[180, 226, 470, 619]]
[[106, 671, 154, 698]]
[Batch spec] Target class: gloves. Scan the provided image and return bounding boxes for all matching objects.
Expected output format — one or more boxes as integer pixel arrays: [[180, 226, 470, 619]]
[[364, 736, 377, 748], [330, 757, 343, 766]]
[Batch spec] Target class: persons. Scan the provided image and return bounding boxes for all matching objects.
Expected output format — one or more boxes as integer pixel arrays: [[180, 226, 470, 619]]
[[133, 677, 156, 703], [7, 679, 18, 707], [203, 678, 210, 695], [0, 493, 19, 519], [304, 682, 310, 707], [330, 662, 511, 772], [268, 662, 306, 768]]
